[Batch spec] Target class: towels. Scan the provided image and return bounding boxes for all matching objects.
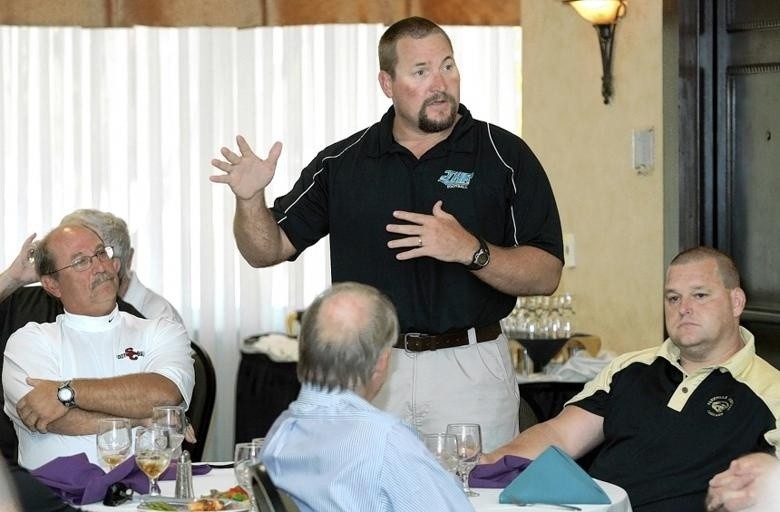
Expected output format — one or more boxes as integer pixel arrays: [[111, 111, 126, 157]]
[[507, 338, 525, 373], [73, 456, 213, 507], [254, 334, 299, 363], [544, 350, 617, 382], [546, 333, 601, 365], [499, 446, 613, 504], [468, 455, 534, 489], [31, 451, 105, 499]]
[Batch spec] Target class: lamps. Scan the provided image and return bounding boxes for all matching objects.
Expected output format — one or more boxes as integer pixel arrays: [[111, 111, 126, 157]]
[[562, 0, 628, 104]]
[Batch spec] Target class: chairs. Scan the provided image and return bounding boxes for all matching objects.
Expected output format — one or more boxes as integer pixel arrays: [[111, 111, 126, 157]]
[[182, 340, 216, 463]]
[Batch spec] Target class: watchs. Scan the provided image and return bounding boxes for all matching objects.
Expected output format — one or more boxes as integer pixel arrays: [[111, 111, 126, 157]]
[[56, 379, 78, 409], [464, 234, 491, 271]]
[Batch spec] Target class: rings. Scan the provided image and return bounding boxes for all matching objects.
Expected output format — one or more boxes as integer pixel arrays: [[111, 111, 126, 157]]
[[417, 237, 423, 247]]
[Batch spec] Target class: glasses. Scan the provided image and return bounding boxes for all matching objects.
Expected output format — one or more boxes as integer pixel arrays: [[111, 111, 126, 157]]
[[104, 482, 134, 506], [48, 245, 115, 272]]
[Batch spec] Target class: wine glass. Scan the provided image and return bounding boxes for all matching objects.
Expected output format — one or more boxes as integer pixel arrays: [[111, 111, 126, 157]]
[[154, 406, 185, 460], [426, 434, 453, 473], [136, 429, 170, 495], [252, 438, 267, 466], [96, 417, 130, 470], [502, 296, 575, 340], [234, 442, 261, 512], [446, 423, 480, 498]]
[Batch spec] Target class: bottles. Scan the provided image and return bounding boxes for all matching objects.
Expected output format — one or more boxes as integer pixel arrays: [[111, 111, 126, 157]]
[[183, 452, 194, 499], [174, 452, 190, 499]]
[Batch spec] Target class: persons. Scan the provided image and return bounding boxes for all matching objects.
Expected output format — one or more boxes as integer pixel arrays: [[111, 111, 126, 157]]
[[465, 245, 780, 511], [207, 16, 565, 456], [256, 283, 477, 512], [0, 223, 196, 480], [0, 207, 185, 329], [707, 451, 780, 511]]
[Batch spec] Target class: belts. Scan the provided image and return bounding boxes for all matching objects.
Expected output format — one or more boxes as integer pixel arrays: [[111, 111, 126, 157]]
[[393, 320, 503, 353]]
